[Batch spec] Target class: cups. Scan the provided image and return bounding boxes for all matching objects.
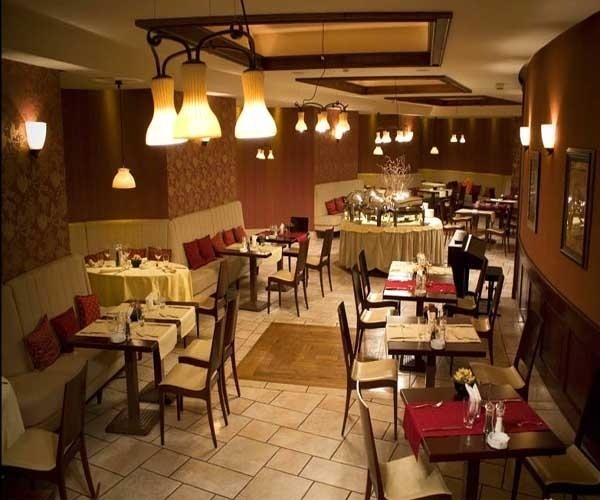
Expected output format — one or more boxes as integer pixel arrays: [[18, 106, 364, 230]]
[[417, 317, 427, 340], [463, 397, 477, 429], [464, 429, 475, 449], [163, 255, 169, 268]]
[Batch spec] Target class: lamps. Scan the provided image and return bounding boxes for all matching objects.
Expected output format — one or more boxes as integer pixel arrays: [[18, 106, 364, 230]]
[[519, 126, 530, 150], [112, 79, 135, 189], [145, 23, 278, 147], [540, 123, 555, 154], [294, 96, 466, 159], [256, 145, 275, 161], [25, 121, 47, 160]]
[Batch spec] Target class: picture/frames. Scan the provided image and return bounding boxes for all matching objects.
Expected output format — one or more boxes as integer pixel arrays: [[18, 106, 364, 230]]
[[559, 148, 595, 269], [526, 150, 541, 234]]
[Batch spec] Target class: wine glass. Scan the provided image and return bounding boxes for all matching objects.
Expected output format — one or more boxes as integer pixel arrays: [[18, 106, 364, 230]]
[[104, 249, 111, 267], [154, 248, 162, 270]]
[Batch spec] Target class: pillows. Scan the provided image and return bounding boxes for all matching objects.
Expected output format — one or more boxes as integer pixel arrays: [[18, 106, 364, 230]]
[[23, 293, 101, 372]]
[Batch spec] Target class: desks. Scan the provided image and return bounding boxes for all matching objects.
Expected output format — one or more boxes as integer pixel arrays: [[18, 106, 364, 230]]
[[70, 299, 200, 436]]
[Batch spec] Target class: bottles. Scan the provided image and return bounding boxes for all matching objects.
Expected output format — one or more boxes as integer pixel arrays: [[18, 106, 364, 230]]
[[412, 266, 428, 289], [485, 400, 495, 433], [113, 316, 119, 332], [430, 319, 438, 339], [440, 318, 447, 341], [495, 400, 506, 433], [116, 248, 122, 267]]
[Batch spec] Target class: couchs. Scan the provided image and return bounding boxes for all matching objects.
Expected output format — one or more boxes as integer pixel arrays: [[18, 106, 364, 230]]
[[0, 253, 125, 449]]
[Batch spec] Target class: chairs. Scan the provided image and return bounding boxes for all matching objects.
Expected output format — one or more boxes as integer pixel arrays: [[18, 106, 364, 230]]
[[178, 290, 241, 414], [0, 360, 97, 500], [157, 295, 228, 449]]
